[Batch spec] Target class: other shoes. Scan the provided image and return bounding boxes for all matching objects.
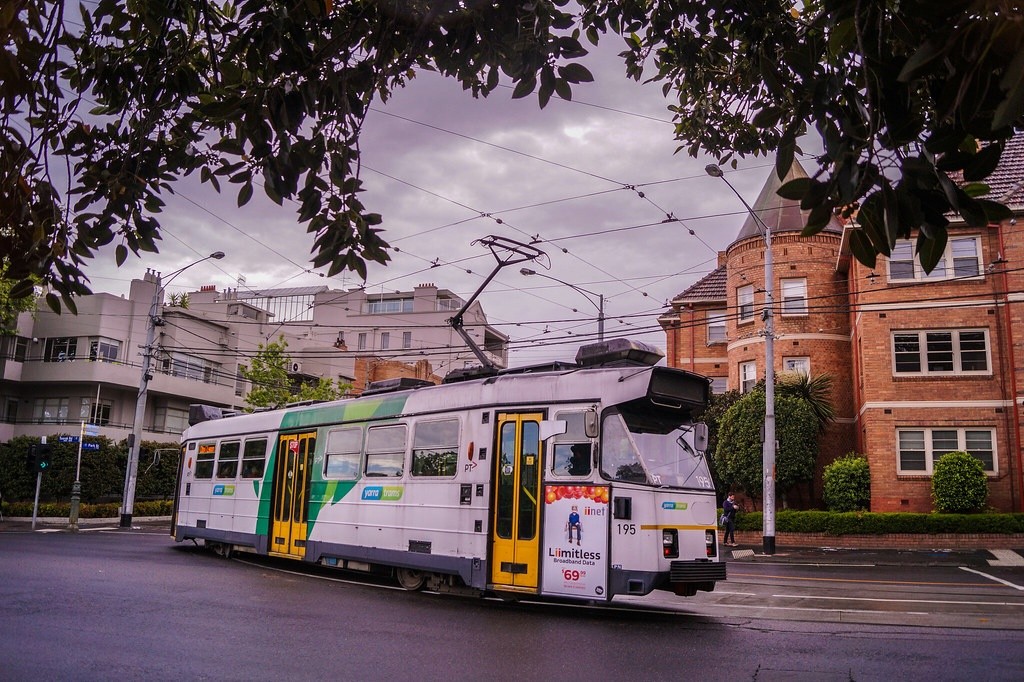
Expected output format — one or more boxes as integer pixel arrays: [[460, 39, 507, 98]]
[[723, 542, 729, 546], [729, 543, 738, 547]]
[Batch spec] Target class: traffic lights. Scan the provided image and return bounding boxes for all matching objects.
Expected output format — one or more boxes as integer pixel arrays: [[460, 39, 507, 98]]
[[35, 444, 53, 473]]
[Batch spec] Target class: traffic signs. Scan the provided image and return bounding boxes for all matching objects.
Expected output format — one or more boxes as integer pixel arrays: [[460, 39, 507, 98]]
[[57, 436, 80, 443], [83, 443, 102, 451]]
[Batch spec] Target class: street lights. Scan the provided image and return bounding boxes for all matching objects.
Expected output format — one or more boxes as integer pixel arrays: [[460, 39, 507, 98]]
[[520, 267, 605, 343], [707, 164, 777, 554], [120, 251, 224, 529]]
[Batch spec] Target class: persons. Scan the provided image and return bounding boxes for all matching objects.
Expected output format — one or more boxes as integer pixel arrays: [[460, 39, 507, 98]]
[[722, 492, 740, 546]]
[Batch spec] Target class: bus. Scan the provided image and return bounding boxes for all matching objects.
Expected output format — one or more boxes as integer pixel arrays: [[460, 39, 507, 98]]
[[169, 338, 726, 604]]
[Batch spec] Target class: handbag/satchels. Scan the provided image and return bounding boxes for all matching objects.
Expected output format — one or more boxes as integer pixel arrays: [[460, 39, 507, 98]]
[[719, 514, 728, 525]]
